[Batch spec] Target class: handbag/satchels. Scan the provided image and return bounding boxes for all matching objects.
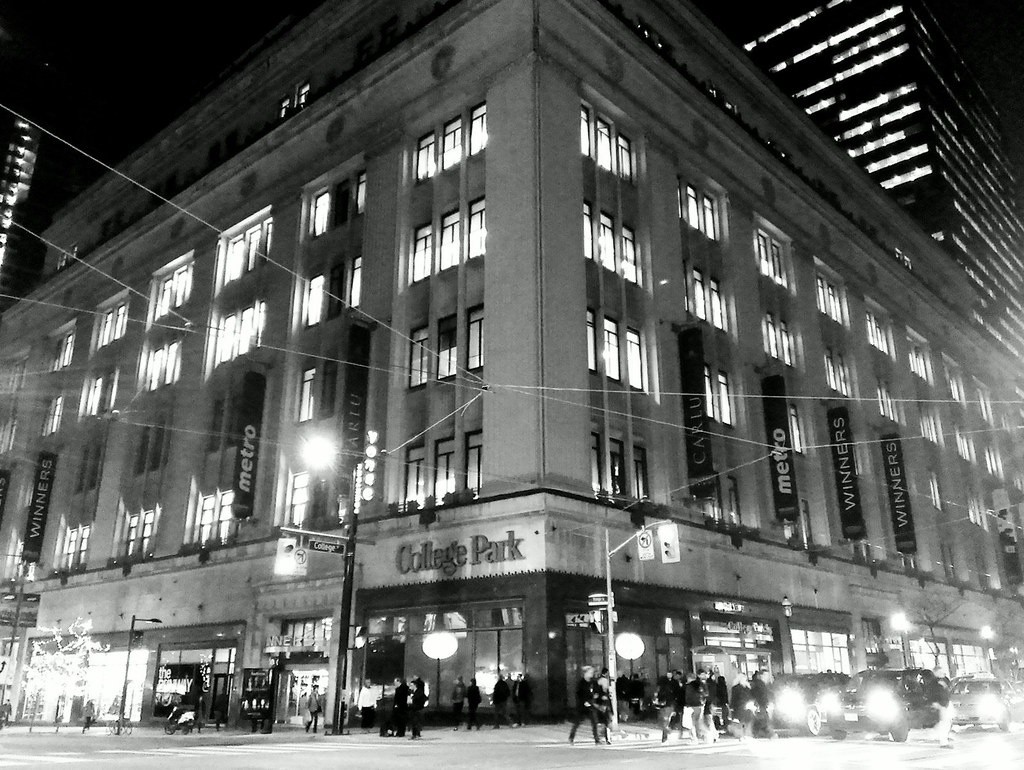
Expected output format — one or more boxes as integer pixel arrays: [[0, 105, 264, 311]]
[[681, 706, 695, 730], [303, 710, 311, 725], [314, 706, 322, 714]]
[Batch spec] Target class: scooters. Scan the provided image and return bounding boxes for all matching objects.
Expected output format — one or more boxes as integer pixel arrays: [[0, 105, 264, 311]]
[[164, 705, 195, 735]]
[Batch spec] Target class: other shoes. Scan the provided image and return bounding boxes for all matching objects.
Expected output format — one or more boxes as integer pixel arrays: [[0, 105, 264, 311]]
[[568, 738, 573, 744], [595, 740, 602, 743], [407, 734, 421, 741]]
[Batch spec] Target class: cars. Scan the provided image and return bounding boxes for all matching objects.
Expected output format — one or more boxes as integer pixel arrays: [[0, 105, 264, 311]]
[[741, 669, 1024, 742]]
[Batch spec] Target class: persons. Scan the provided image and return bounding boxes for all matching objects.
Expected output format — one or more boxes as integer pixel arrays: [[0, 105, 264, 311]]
[[84, 700, 95, 729], [305, 685, 320, 733], [4, 699, 12, 722], [213, 687, 228, 731], [492, 672, 534, 729], [451, 676, 465, 731], [189, 695, 206, 733], [616, 671, 645, 723], [568, 665, 611, 744], [465, 678, 481, 730], [652, 665, 777, 743], [358, 678, 377, 734], [389, 675, 425, 739]]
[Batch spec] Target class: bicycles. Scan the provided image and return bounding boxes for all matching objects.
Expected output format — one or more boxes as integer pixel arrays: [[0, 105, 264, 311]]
[[109, 712, 133, 736]]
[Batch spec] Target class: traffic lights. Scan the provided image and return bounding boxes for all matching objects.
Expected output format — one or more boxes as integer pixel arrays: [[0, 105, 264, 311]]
[[274, 537, 297, 577], [656, 523, 682, 564], [354, 625, 368, 650], [589, 610, 608, 636]]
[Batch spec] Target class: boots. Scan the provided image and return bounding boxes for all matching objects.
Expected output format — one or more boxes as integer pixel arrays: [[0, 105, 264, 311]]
[[605, 727, 612, 745]]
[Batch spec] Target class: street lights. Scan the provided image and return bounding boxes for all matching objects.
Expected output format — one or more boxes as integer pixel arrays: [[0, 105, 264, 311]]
[[979, 625, 995, 674], [297, 434, 361, 736], [780, 596, 798, 673], [118, 614, 163, 735], [889, 611, 910, 669]]
